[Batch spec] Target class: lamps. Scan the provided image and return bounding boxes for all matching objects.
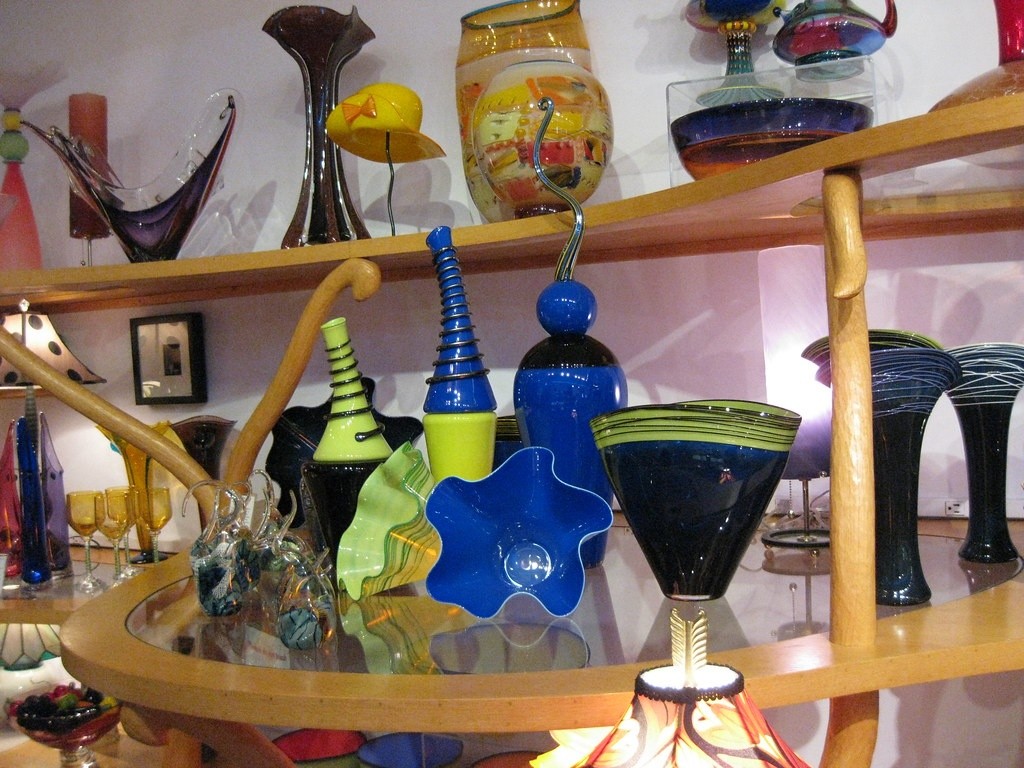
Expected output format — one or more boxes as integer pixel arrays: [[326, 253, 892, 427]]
[[572, 608, 819, 768], [0, 622, 63, 671], [0, 298, 107, 461]]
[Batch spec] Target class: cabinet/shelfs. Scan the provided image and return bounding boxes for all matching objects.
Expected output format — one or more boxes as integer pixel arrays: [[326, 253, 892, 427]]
[[0, 84, 1023, 768]]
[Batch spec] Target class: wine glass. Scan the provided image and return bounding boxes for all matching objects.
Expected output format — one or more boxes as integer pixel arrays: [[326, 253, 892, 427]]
[[93, 492, 131, 581], [3, 677, 120, 768], [137, 487, 173, 563], [66, 491, 105, 594], [105, 486, 142, 580]]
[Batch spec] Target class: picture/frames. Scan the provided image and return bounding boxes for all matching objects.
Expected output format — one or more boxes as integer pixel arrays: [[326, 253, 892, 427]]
[[129, 312, 208, 405]]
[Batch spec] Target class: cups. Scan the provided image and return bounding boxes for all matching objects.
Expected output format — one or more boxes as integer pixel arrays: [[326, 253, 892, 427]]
[[590, 401, 802, 602]]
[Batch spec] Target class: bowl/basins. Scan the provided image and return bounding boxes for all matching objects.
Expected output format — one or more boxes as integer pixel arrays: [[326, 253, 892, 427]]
[[669, 98, 874, 182]]
